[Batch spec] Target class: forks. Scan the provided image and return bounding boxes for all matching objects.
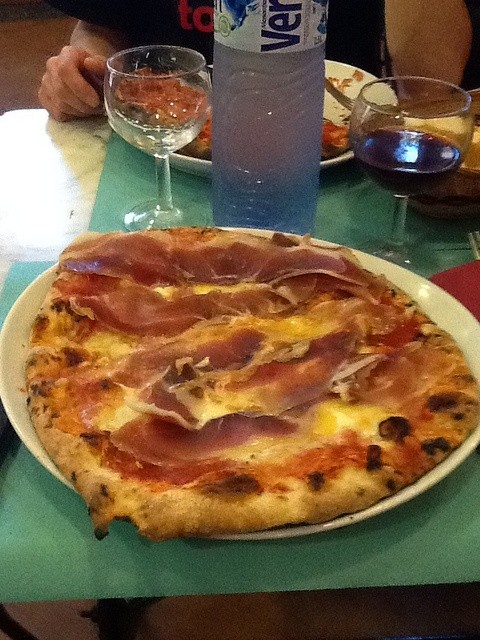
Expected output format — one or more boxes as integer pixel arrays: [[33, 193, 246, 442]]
[[324, 78, 479, 113]]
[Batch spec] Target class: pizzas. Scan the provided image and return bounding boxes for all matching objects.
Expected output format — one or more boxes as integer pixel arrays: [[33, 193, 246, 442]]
[[107, 68, 355, 163]]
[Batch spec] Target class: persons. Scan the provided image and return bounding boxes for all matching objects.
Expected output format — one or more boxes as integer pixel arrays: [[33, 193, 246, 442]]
[[37, 1, 480, 122]]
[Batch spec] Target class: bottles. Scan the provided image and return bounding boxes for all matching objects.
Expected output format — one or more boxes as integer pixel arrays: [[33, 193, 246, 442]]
[[212, 0, 328, 237]]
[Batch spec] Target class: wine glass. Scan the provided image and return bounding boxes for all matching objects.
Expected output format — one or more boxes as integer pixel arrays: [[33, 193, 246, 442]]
[[349, 75, 474, 269], [104, 46, 211, 231]]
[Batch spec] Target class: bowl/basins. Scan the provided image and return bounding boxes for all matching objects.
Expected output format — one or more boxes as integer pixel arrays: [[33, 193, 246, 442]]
[[368, 97, 480, 221]]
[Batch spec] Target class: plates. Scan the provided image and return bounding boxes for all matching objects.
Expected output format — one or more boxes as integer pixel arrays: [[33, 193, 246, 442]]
[[115, 60, 399, 179]]
